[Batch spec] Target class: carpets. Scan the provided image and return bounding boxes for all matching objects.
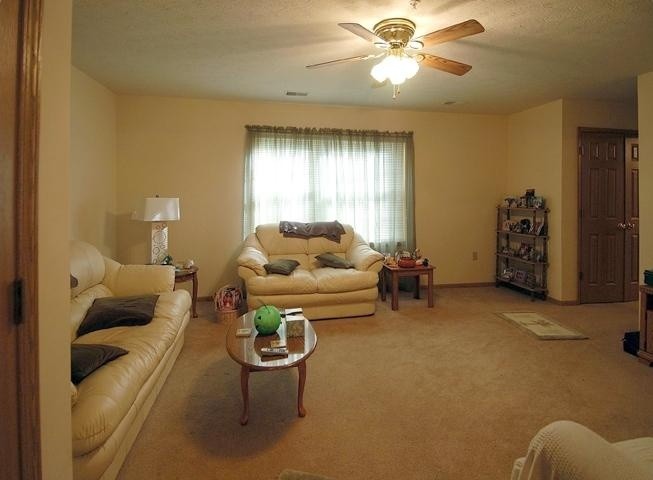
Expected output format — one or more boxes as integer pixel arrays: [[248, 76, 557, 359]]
[[492, 310, 591, 341]]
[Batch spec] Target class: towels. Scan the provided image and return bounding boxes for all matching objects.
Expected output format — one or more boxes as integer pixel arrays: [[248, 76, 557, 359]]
[[279, 222, 347, 244]]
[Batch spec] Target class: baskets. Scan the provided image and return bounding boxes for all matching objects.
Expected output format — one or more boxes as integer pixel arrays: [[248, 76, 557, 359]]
[[214, 285, 242, 323]]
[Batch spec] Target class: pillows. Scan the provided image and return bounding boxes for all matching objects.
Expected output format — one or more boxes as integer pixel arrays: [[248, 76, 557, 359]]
[[71, 342, 130, 387], [313, 252, 353, 269], [75, 291, 160, 339], [264, 259, 301, 277]]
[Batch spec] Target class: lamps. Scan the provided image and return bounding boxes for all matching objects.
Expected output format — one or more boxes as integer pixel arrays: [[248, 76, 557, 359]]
[[371, 49, 420, 100], [142, 193, 181, 265]]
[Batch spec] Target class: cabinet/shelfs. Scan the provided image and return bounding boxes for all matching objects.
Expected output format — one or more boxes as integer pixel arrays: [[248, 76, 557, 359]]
[[638, 284, 653, 365], [494, 206, 551, 302]]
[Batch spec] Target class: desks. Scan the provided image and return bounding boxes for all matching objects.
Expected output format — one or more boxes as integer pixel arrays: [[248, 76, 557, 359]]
[[381, 259, 436, 312], [145, 262, 199, 319]]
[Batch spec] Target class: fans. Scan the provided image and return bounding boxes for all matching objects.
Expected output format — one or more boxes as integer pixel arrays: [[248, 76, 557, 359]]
[[304, 17, 486, 79]]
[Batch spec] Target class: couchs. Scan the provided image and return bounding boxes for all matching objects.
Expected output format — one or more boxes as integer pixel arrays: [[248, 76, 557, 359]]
[[70, 240, 195, 480], [236, 221, 387, 321], [510, 420, 653, 479]]
